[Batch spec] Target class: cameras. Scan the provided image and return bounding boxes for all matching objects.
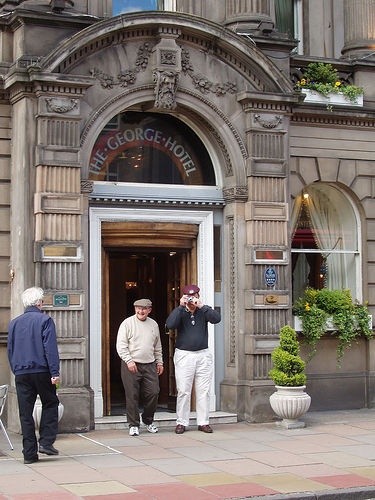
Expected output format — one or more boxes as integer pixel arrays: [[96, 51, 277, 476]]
[[186, 297, 193, 302]]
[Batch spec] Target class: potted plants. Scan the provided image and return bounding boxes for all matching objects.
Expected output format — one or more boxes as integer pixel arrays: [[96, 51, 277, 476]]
[[292, 285, 375, 369], [32, 379, 65, 442], [266, 323, 312, 429]]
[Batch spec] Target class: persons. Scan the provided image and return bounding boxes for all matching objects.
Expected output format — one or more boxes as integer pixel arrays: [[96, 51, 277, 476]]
[[116, 299, 164, 435], [166, 285, 222, 434], [7, 285, 60, 464]]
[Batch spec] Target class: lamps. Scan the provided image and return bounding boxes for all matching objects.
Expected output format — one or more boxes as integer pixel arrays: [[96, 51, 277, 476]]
[[257, 20, 275, 37], [49, 0, 66, 13], [302, 189, 309, 199]]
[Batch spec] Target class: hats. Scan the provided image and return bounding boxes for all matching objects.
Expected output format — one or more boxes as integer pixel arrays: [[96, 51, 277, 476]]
[[134, 299, 152, 307], [182, 285, 200, 294]]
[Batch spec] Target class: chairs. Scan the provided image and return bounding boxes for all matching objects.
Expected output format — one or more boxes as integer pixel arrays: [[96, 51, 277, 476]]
[[0, 383, 15, 451]]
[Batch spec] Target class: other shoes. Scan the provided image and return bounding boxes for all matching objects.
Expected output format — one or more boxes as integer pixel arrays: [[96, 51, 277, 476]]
[[129, 427, 139, 436], [24, 454, 39, 464], [175, 424, 185, 434], [141, 422, 158, 433], [39, 445, 59, 455], [198, 425, 213, 433]]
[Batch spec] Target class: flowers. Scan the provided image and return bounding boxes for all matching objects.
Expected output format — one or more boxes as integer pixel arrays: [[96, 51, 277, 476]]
[[294, 61, 365, 113]]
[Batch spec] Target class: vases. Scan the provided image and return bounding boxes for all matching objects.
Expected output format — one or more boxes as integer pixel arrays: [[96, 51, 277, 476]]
[[300, 88, 364, 108]]
